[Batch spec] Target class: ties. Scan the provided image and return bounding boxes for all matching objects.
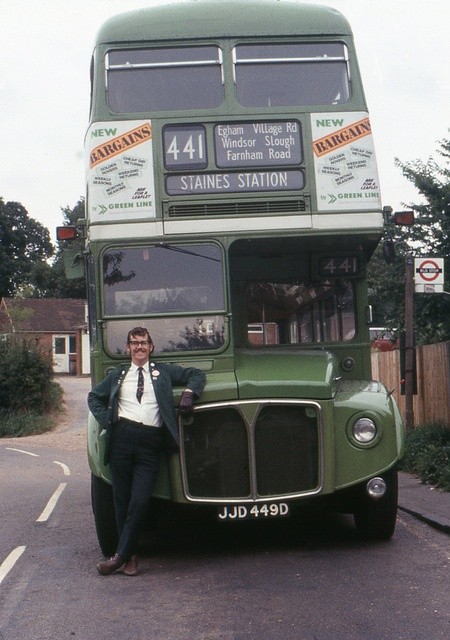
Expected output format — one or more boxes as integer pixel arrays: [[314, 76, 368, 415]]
[[137, 367, 143, 404]]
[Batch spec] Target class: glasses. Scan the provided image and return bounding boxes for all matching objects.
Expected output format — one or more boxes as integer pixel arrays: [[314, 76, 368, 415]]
[[128, 341, 151, 345]]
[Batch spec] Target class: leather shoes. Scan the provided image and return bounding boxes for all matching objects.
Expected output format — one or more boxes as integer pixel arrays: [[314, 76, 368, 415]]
[[124, 555, 137, 575], [97, 553, 123, 575]]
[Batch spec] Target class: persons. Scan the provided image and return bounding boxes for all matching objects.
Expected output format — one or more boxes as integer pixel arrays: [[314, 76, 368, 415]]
[[87, 328, 206, 576]]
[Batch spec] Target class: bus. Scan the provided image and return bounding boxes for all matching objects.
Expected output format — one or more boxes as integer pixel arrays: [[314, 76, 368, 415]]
[[55, 1, 416, 564]]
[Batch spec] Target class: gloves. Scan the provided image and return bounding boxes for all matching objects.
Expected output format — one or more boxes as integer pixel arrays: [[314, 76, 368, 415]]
[[179, 392, 193, 418]]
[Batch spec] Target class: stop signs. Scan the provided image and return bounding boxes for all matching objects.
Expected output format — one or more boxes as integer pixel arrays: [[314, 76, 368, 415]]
[[413, 256, 445, 295]]
